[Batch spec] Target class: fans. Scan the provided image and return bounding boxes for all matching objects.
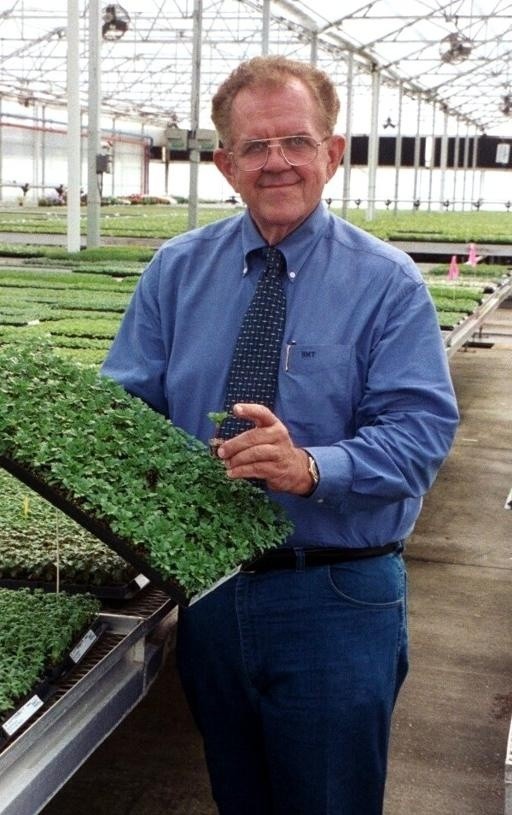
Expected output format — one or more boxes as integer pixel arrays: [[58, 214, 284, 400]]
[[439, 31, 472, 66], [102, 3, 131, 40]]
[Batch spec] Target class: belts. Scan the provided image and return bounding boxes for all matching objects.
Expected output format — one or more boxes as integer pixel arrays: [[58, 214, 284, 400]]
[[237, 540, 403, 577]]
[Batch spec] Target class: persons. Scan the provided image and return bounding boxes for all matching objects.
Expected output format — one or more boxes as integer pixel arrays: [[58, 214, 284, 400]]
[[100, 50, 463, 815]]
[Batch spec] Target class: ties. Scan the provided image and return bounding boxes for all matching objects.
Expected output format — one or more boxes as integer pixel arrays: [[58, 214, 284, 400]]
[[216, 246, 286, 487]]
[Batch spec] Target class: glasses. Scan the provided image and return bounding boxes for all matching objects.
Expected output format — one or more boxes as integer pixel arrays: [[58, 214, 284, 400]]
[[220, 134, 332, 174]]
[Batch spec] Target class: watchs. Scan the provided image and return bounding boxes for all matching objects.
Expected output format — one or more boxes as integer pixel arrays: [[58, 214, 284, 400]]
[[299, 456, 320, 497]]
